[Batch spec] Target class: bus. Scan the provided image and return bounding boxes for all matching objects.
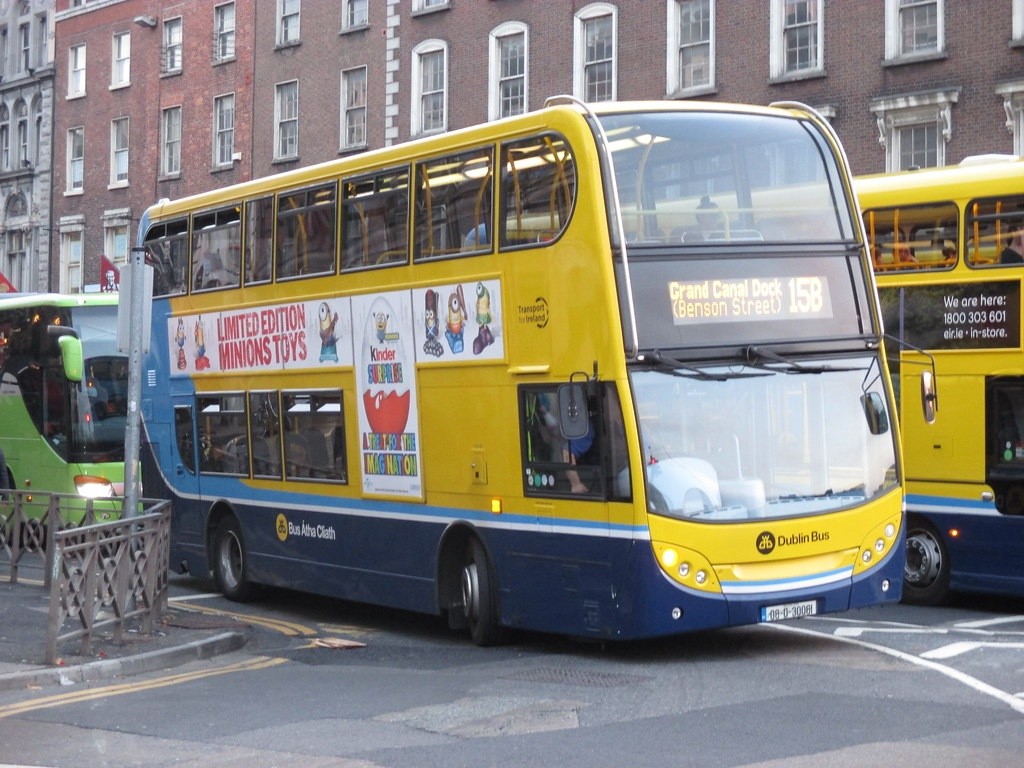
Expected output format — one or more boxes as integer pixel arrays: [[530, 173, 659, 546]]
[[463, 154, 1024, 603], [32, 338, 129, 465], [1, 293, 143, 549], [139, 94, 940, 648]]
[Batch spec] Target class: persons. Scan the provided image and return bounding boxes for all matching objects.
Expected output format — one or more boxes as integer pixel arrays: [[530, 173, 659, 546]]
[[1002, 218, 1024, 264], [891, 246, 920, 270], [691, 197, 721, 241], [941, 244, 956, 268], [563, 393, 653, 497]]
